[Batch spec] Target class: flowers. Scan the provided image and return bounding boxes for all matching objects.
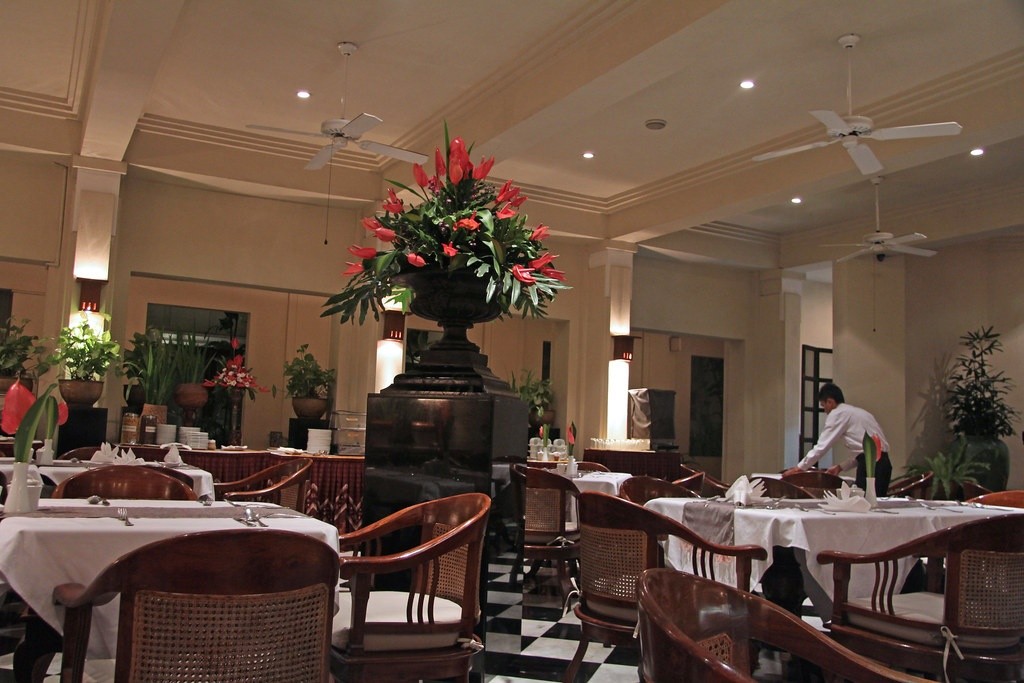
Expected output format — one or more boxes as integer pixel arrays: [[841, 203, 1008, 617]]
[[861, 428, 883, 478], [538, 424, 549, 447], [567, 420, 577, 457], [43, 394, 69, 440], [2, 375, 60, 464], [315, 113, 572, 322]]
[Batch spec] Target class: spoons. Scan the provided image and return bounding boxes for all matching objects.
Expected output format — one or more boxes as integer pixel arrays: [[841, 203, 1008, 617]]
[[694, 491, 1014, 517], [87, 495, 101, 503]]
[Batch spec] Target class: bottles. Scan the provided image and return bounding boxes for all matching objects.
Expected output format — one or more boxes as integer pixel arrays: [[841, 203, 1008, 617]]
[[140, 414, 158, 444], [120, 413, 138, 442], [209, 439, 216, 450]]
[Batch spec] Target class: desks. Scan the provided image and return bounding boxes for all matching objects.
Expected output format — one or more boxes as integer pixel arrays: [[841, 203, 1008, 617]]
[[583, 449, 683, 484], [115, 439, 366, 549]]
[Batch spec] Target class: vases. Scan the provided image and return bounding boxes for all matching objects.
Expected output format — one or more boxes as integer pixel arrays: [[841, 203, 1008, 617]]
[[4, 460, 34, 514], [227, 386, 247, 447], [867, 477, 880, 509], [399, 262, 506, 379], [543, 447, 548, 462], [40, 436, 55, 465]]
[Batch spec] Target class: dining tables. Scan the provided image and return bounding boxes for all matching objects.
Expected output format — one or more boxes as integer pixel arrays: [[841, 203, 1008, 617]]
[[2, 496, 345, 683], [644, 494, 1024, 683], [3, 455, 216, 503]]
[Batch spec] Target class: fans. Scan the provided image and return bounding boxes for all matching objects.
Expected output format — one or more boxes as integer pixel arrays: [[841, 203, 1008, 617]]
[[816, 171, 939, 334], [746, 32, 965, 180], [242, 40, 430, 250]]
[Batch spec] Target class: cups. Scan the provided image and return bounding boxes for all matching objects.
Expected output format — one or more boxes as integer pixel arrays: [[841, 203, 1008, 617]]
[[539, 449, 579, 476], [246, 503, 260, 521], [590, 438, 651, 451]]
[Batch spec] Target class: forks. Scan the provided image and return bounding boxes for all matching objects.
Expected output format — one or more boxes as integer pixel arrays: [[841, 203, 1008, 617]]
[[202, 498, 213, 505], [118, 508, 132, 527]]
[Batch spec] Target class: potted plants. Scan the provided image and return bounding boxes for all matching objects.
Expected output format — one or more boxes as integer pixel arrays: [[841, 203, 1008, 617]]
[[904, 441, 993, 500], [0, 313, 337, 416], [528, 379, 557, 427], [937, 318, 1023, 499]]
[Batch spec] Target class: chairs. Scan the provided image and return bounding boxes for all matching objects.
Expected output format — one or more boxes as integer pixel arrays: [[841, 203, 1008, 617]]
[[326, 492, 493, 683], [508, 462, 584, 612], [637, 564, 947, 682], [50, 526, 340, 682], [213, 455, 316, 514], [816, 514, 1024, 682], [558, 491, 771, 682], [515, 454, 1024, 538], [0, 442, 199, 505]]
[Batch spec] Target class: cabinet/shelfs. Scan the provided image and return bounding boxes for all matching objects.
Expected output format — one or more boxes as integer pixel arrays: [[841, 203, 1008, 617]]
[[287, 417, 329, 451], [527, 425, 560, 449], [58, 405, 108, 461]]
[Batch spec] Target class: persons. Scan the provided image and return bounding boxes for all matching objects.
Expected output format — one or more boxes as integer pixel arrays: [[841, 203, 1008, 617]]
[[784, 383, 892, 499]]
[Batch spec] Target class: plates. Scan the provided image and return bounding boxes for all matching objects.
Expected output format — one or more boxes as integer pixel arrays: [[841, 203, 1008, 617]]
[[144, 444, 160, 446], [307, 429, 332, 455], [122, 442, 141, 446], [155, 423, 209, 449], [223, 445, 248, 450]]
